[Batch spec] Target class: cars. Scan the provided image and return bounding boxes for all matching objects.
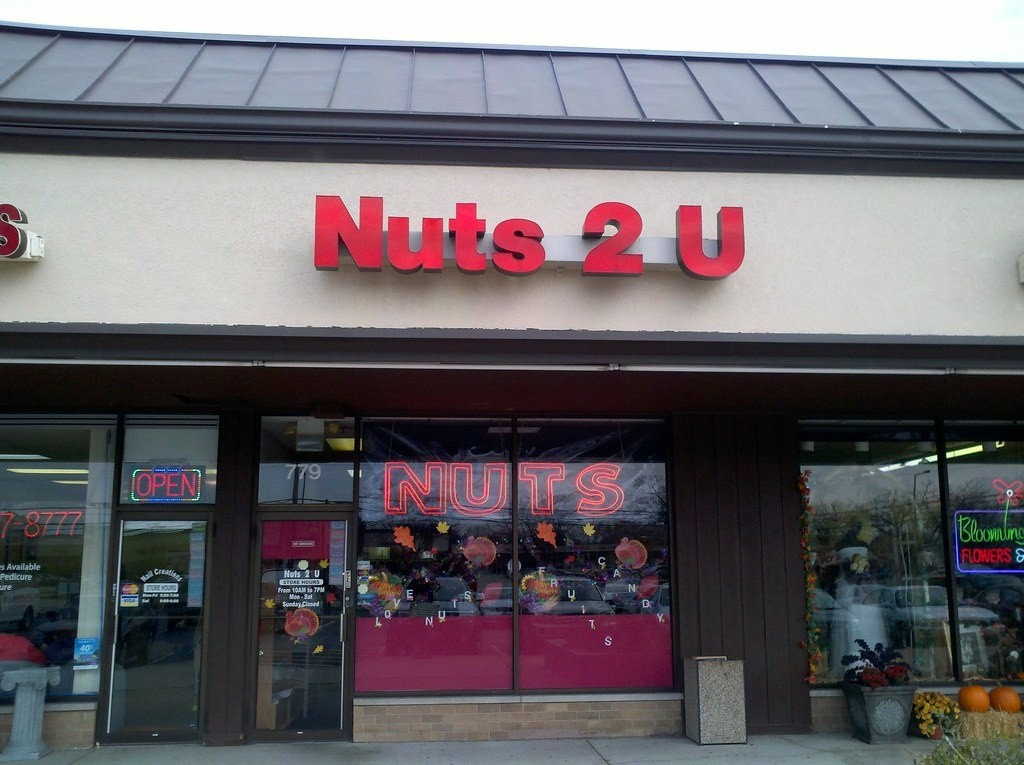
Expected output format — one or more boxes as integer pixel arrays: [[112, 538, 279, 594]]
[[646, 583, 671, 618], [526, 573, 616, 616], [604, 583, 642, 614], [866, 584, 1005, 645], [481, 586, 526, 615], [35, 594, 160, 665]]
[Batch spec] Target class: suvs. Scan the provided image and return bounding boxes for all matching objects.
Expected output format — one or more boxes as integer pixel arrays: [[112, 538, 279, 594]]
[[397, 574, 485, 616]]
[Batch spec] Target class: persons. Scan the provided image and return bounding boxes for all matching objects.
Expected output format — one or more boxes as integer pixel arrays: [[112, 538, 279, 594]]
[[0, 619, 45, 663], [825, 530, 888, 678], [27, 630, 55, 664]]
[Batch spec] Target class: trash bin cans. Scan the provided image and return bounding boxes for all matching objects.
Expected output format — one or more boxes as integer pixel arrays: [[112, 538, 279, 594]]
[[684, 655, 748, 745]]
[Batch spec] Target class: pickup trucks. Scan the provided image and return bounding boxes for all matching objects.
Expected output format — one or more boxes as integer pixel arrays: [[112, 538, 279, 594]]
[[0, 587, 66, 631]]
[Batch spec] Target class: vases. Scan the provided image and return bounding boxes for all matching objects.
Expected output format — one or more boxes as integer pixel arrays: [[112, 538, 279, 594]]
[[924, 727, 941, 739], [841, 687, 917, 745]]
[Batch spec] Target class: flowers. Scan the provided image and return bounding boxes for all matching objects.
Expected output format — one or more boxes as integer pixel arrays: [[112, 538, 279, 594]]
[[841, 638, 913, 693], [913, 691, 961, 737]]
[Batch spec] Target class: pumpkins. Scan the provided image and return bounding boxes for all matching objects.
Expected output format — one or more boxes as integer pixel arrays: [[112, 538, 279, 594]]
[[988, 681, 1021, 714], [957, 683, 991, 713]]
